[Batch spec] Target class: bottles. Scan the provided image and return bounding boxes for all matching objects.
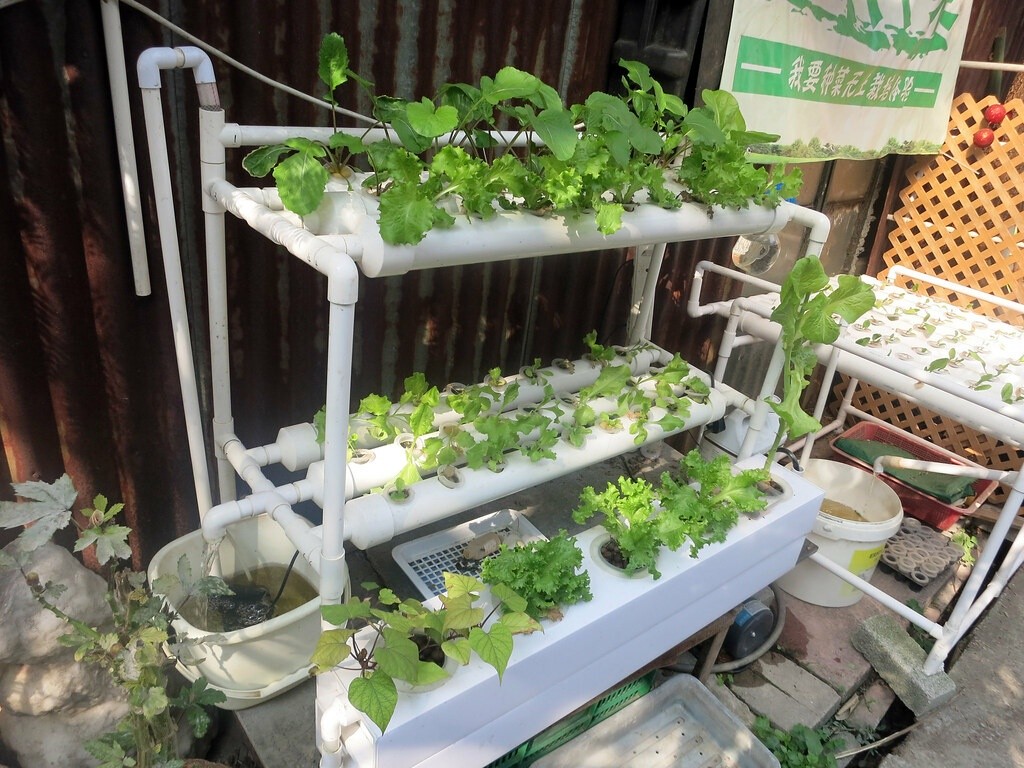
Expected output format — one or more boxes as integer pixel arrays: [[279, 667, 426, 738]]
[[463, 532, 502, 561], [700, 392, 788, 466]]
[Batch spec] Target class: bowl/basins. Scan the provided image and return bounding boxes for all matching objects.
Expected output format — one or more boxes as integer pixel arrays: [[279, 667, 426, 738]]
[[663, 578, 787, 687]]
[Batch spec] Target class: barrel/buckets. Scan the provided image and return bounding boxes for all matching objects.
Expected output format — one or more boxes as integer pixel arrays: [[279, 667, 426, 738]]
[[777, 458, 904, 609], [146, 513, 352, 710]]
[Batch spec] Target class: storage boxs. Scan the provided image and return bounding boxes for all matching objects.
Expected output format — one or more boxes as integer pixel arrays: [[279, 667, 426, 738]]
[[483, 669, 782, 768], [829, 420, 1000, 530]]
